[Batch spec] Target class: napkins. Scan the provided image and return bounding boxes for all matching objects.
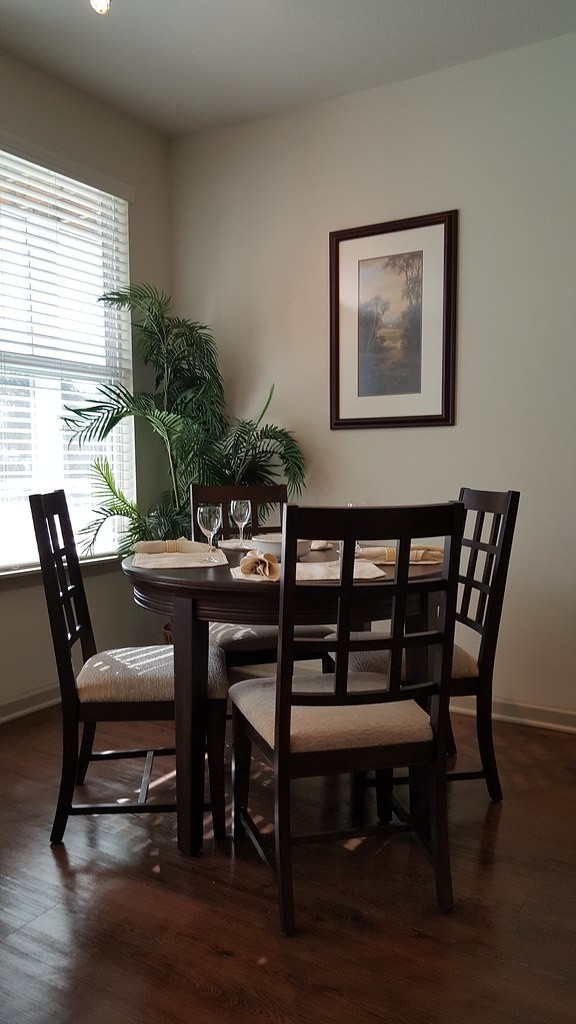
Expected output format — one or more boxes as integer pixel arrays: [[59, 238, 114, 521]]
[[130, 535, 211, 553], [376, 544, 445, 561], [237, 549, 281, 582]]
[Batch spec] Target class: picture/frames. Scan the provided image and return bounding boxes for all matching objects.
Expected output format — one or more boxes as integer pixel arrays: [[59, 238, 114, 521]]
[[328, 209, 459, 431]]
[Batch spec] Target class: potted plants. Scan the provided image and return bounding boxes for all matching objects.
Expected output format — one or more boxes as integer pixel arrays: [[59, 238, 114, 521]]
[[60, 281, 309, 644]]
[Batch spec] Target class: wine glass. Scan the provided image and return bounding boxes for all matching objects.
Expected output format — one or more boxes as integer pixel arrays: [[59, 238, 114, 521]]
[[347, 500, 369, 553], [196, 506, 223, 564], [230, 500, 252, 548]]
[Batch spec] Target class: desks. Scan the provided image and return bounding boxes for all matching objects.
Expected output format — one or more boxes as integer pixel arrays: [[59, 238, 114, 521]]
[[121, 543, 447, 855]]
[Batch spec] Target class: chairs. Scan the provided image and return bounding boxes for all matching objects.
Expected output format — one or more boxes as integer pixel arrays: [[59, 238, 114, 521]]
[[28, 488, 231, 845], [187, 482, 338, 762], [325, 487, 521, 824], [227, 501, 468, 936]]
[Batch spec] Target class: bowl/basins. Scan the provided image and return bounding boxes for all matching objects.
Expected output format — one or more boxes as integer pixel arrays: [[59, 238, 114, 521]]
[[251, 533, 311, 561]]
